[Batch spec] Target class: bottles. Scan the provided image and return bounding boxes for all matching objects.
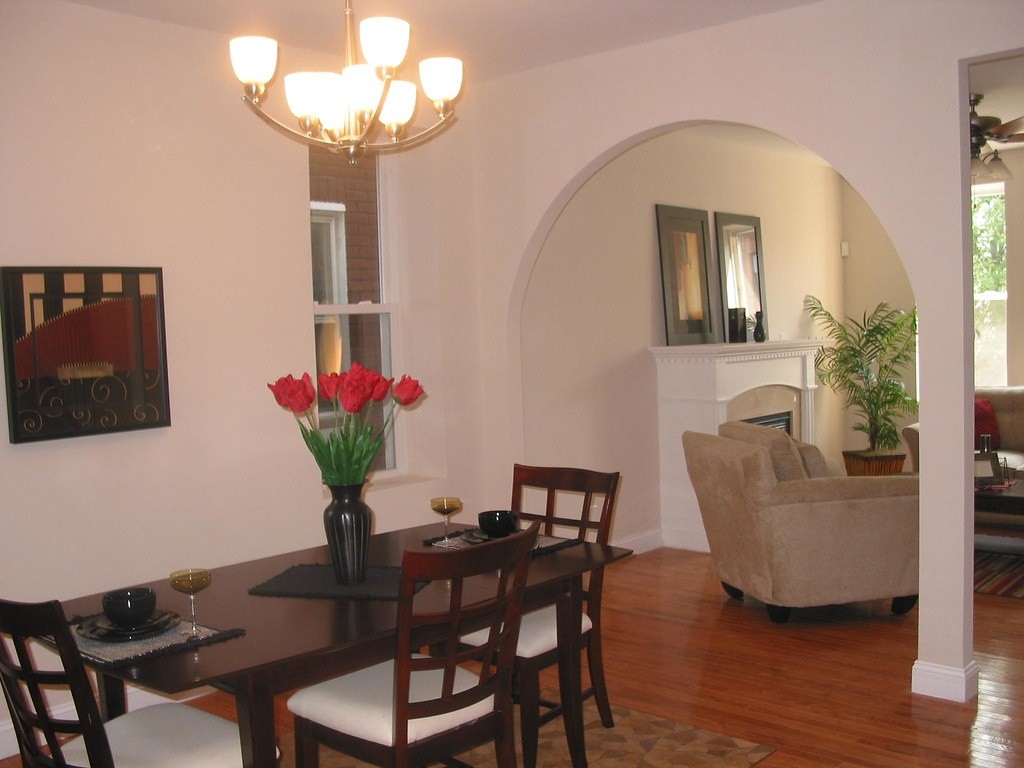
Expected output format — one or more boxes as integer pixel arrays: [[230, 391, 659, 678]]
[[754, 311, 765, 342]]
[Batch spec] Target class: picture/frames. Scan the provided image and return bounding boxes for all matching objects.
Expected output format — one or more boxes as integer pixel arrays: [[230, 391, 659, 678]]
[[714, 212, 768, 342], [656, 205, 719, 346], [0, 266, 170, 444]]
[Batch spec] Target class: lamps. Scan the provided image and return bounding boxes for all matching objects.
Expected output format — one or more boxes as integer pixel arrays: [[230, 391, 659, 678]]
[[229, 0, 464, 166], [972, 137, 1013, 181]]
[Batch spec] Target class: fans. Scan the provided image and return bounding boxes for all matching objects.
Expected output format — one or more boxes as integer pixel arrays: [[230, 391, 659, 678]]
[[969, 94, 1024, 145]]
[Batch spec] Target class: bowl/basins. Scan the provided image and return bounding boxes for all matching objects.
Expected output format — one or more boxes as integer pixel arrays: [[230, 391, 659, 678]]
[[102, 587, 157, 624], [479, 511, 515, 537]]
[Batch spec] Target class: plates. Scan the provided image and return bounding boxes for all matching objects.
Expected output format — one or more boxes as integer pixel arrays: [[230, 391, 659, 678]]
[[460, 529, 524, 544], [76, 611, 182, 642]]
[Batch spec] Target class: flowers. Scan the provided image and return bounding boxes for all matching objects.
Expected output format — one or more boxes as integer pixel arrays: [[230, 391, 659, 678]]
[[266, 360, 424, 484]]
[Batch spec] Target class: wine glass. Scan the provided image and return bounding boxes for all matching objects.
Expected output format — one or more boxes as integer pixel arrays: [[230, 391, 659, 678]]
[[170, 569, 212, 636], [431, 497, 462, 545]]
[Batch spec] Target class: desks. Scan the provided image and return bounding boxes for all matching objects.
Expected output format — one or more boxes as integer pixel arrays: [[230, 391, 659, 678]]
[[36, 521, 633, 768]]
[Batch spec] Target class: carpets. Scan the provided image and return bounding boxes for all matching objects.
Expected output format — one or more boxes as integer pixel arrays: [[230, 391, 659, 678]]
[[280, 686, 778, 768], [974, 548, 1024, 598]]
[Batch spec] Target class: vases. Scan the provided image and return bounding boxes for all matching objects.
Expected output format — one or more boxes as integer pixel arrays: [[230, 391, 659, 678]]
[[322, 479, 373, 584]]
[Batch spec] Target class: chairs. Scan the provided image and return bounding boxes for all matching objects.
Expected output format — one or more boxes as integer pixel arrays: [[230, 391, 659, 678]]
[[0, 600, 281, 768], [287, 519, 540, 768], [458, 465, 620, 768]]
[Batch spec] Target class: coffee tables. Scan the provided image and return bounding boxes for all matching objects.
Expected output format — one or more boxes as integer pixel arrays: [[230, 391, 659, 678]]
[[975, 477, 1024, 516]]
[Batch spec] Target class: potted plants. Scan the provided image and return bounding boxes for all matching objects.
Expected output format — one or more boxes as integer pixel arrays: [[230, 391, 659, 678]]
[[804, 294, 920, 475]]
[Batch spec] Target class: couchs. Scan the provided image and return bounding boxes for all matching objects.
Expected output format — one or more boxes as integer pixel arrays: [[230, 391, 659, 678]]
[[902, 387, 1024, 538], [681, 421, 919, 623]]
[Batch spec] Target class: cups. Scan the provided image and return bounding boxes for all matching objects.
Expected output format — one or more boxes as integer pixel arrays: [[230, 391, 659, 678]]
[[981, 434, 991, 453]]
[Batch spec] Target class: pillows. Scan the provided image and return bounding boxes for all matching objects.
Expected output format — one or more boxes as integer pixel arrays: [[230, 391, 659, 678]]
[[974, 397, 1000, 451]]
